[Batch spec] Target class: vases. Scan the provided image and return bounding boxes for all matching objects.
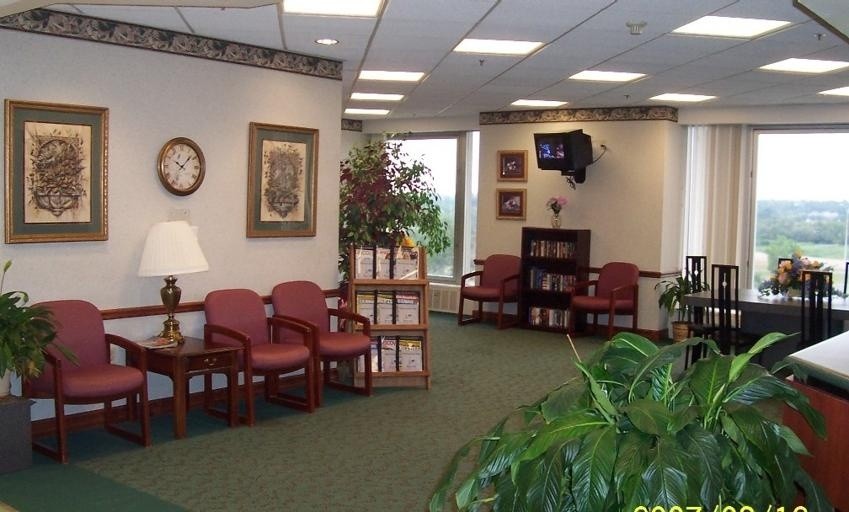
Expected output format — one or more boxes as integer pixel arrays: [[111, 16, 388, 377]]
[[550, 213, 562, 230]]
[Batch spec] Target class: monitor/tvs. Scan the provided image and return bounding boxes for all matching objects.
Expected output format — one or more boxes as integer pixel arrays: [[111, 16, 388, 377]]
[[534, 128, 593, 171]]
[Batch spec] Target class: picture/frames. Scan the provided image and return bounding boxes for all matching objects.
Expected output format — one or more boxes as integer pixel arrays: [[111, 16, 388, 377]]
[[245, 121, 320, 239], [2, 98, 110, 245], [495, 147, 529, 183], [493, 187, 527, 224]]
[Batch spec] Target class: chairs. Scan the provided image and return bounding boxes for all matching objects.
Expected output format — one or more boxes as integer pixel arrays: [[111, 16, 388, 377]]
[[456, 252, 523, 331], [19, 299, 149, 472], [564, 262, 643, 341], [683, 255, 741, 368], [204, 288, 319, 431], [799, 269, 833, 350], [267, 278, 374, 405], [708, 264, 766, 366]]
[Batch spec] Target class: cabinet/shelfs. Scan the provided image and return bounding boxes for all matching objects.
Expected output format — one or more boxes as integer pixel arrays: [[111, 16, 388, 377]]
[[520, 228, 591, 333], [338, 239, 433, 391]]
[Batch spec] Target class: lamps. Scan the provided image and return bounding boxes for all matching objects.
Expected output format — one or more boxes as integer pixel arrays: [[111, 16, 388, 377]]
[[136, 219, 212, 342]]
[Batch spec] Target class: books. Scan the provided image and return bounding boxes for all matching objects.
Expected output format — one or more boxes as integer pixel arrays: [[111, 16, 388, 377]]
[[356, 247, 423, 373], [527, 238, 575, 328]]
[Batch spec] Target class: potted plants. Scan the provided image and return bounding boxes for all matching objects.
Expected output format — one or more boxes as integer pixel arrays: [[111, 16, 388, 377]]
[[653, 276, 711, 345], [0, 257, 84, 475]]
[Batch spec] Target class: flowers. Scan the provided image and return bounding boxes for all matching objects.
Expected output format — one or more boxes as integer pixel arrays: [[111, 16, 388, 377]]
[[545, 193, 569, 215], [756, 245, 848, 301]]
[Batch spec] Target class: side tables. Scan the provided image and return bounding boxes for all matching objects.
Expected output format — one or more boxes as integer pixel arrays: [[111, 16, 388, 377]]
[[126, 332, 244, 440]]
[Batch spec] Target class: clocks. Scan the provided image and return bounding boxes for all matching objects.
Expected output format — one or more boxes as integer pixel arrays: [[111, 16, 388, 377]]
[[153, 135, 209, 197]]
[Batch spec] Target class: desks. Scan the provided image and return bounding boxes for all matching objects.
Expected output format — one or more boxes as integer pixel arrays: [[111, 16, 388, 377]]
[[680, 285, 849, 363]]
